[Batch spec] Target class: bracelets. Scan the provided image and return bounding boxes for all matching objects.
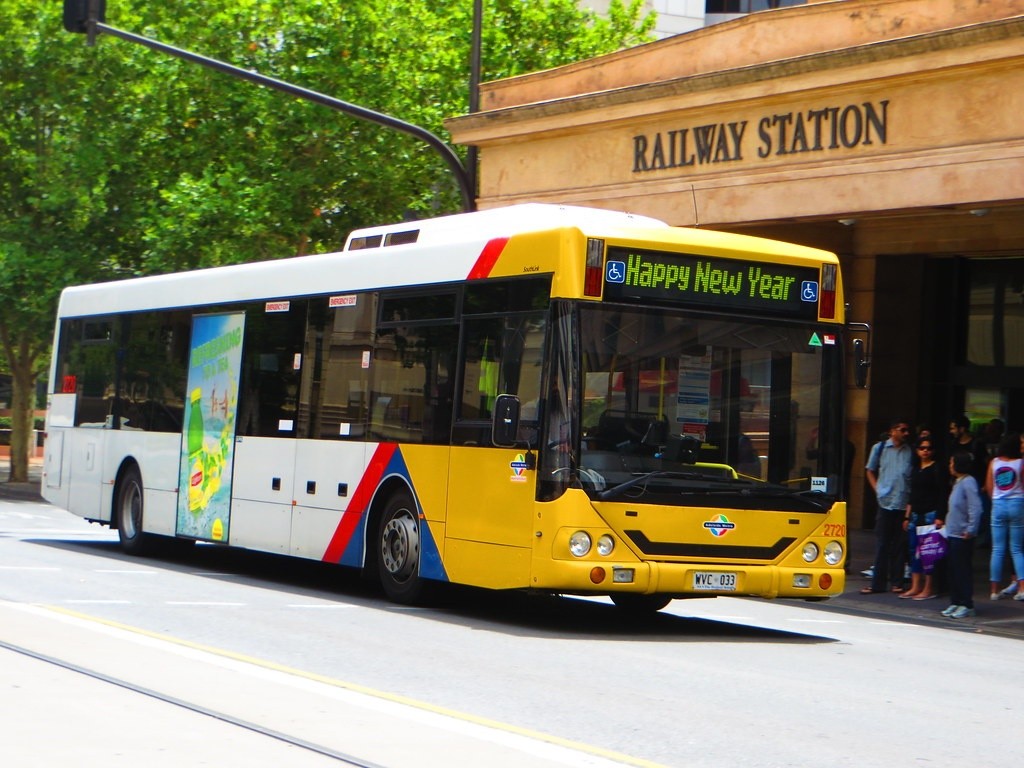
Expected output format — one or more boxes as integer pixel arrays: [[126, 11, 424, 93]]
[[903, 517, 909, 521]]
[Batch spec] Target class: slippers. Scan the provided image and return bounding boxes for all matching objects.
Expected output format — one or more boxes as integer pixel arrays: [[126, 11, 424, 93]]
[[898, 592, 912, 598], [861, 586, 874, 595], [912, 594, 930, 601], [892, 587, 904, 593]]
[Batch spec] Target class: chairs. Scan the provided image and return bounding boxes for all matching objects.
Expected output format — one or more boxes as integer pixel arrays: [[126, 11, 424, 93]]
[[599, 409, 669, 457], [422, 401, 483, 446]]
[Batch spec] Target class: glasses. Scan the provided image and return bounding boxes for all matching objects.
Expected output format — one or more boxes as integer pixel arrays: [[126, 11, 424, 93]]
[[894, 428, 912, 433], [916, 446, 933, 451]]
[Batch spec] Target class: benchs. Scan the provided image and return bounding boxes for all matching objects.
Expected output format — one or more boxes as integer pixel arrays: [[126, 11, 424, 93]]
[[0, 429, 43, 459]]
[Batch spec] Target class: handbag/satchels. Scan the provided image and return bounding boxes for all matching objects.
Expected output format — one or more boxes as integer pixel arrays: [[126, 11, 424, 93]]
[[916, 524, 948, 574]]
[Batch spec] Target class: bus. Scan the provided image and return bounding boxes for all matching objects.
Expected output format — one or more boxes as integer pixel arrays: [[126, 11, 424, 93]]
[[41, 203, 875, 612]]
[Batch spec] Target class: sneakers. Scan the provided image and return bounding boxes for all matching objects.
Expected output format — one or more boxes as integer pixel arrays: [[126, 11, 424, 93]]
[[1000, 581, 1018, 595], [860, 566, 874, 579], [990, 593, 999, 601], [1013, 592, 1024, 600], [940, 605, 975, 619]]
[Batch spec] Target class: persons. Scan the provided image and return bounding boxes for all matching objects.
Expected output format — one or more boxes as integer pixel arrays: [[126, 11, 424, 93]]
[[803, 413, 1024, 621]]
